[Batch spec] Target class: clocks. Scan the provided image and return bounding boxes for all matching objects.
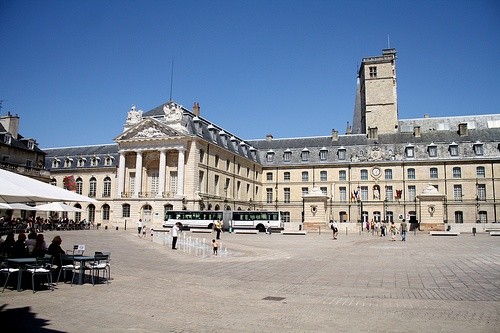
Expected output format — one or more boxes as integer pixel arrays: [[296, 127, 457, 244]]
[[372, 168, 380, 176]]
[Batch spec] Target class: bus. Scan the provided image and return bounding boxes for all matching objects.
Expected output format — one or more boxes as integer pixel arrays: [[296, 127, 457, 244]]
[[162, 209, 290, 233]]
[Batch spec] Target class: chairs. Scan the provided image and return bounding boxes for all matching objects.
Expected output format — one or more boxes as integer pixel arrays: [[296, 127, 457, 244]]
[[0, 221, 111, 294]]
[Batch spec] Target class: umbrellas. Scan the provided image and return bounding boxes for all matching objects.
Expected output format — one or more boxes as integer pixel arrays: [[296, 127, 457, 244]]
[[0, 169, 97, 202], [34, 202, 87, 212], [0, 202, 37, 210]]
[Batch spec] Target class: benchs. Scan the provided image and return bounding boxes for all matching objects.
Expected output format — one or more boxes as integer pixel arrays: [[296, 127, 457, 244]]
[[153, 228, 172, 232], [234, 230, 259, 234], [190, 228, 213, 233], [429, 230, 460, 235], [281, 230, 307, 235], [485, 224, 500, 236]]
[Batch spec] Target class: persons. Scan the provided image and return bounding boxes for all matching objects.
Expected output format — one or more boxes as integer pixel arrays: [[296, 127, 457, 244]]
[[216, 220, 222, 239], [266, 220, 271, 234], [330, 221, 338, 240], [172, 223, 179, 249], [389, 222, 398, 241], [399, 219, 408, 241], [136, 219, 154, 239], [367, 218, 386, 237], [0, 215, 94, 235], [211, 239, 218, 256], [0, 232, 73, 265]]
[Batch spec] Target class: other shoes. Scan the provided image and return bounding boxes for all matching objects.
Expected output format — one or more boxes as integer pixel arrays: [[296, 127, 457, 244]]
[[172, 248, 176, 249]]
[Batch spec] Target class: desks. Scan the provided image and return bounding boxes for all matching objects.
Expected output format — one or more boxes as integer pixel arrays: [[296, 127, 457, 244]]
[[62, 255, 108, 285], [7, 257, 56, 292]]
